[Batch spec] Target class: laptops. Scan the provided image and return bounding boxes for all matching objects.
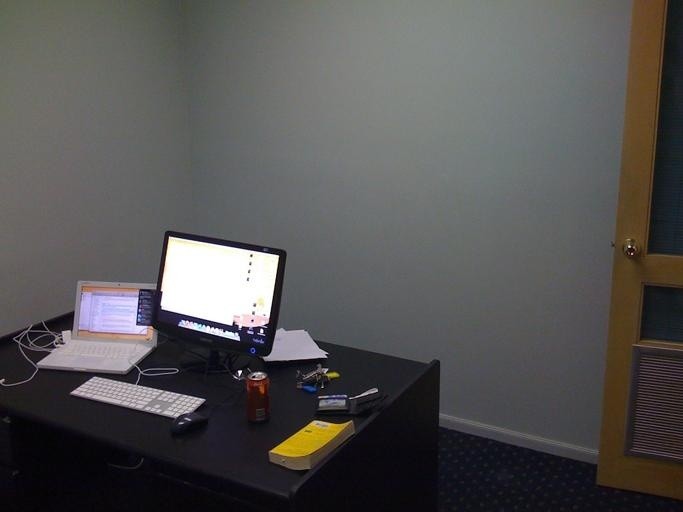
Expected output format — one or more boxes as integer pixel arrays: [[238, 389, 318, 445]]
[[35, 280, 158, 375]]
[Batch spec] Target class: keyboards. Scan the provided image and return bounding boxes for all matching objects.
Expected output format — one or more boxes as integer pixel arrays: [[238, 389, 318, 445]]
[[69, 376, 208, 419]]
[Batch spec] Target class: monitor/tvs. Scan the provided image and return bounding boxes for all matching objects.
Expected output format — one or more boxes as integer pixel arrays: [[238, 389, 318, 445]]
[[151, 229, 287, 373]]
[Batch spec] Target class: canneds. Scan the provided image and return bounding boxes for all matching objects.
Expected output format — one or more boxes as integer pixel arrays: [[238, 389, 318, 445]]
[[245, 371, 271, 424]]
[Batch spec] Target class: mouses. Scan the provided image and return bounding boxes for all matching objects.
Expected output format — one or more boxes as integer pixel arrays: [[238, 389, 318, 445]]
[[169, 412, 207, 437]]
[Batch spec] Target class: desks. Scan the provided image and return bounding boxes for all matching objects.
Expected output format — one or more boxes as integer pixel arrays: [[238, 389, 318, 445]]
[[0, 311, 441, 511]]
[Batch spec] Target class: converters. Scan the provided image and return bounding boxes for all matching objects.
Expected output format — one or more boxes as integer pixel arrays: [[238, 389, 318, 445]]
[[62, 330, 71, 344]]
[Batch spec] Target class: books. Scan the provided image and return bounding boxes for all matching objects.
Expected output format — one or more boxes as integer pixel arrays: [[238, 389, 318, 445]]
[[268, 418, 356, 472]]
